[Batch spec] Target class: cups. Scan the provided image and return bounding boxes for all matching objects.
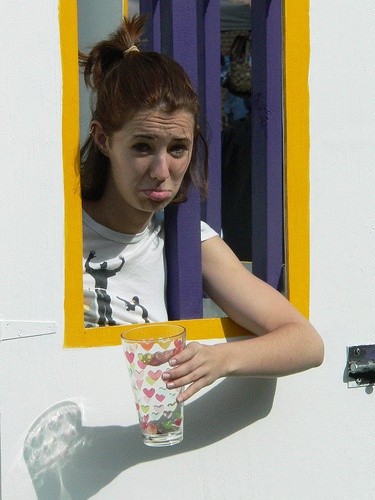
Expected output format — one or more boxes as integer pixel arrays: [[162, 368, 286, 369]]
[[120, 324, 186, 447]]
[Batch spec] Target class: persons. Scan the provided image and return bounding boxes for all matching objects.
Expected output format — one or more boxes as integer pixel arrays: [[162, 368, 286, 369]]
[[78, 16, 324, 402]]
